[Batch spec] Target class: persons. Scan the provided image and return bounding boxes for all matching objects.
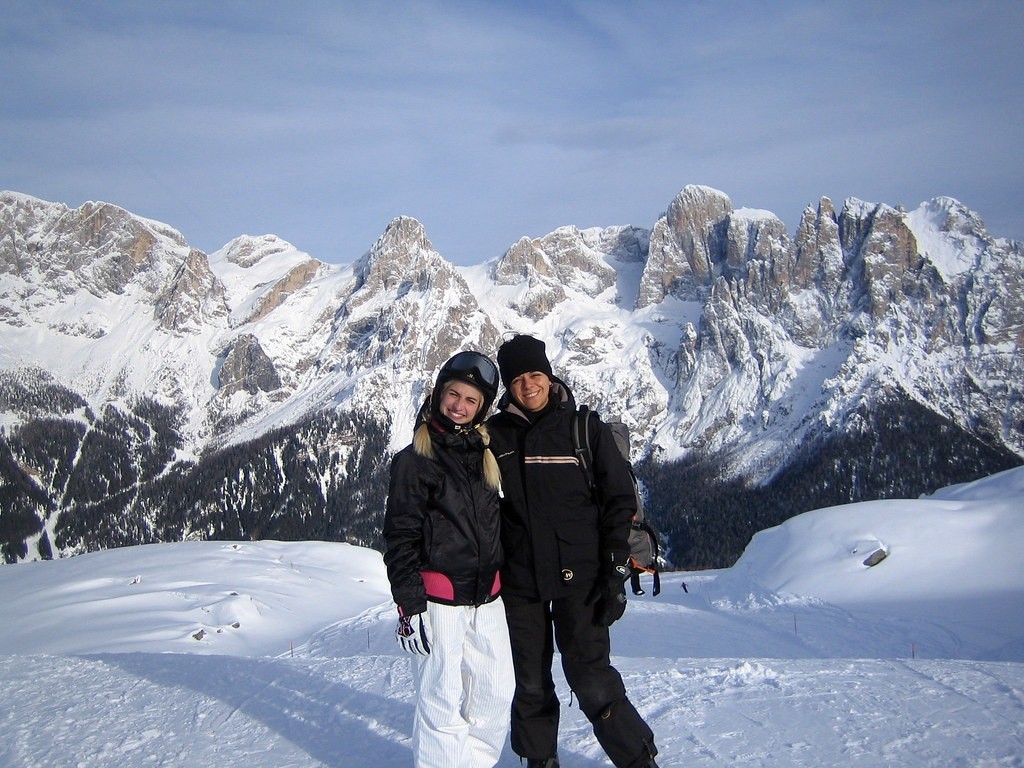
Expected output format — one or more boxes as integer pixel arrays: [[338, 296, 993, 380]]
[[485, 335, 658, 768], [382, 350, 518, 768]]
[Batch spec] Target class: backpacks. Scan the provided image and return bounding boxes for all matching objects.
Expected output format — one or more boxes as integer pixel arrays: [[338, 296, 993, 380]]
[[571, 404, 662, 596]]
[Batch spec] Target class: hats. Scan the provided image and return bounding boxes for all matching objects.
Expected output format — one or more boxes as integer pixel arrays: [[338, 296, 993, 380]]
[[497, 335, 554, 394]]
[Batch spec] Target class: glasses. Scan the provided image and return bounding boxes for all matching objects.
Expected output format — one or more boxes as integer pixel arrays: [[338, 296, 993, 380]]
[[448, 353, 495, 385]]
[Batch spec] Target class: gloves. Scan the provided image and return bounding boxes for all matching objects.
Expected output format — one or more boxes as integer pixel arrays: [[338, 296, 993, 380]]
[[394, 611, 433, 657], [583, 547, 631, 628]]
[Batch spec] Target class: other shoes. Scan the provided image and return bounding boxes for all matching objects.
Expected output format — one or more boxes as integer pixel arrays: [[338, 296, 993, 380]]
[[527, 753, 560, 768]]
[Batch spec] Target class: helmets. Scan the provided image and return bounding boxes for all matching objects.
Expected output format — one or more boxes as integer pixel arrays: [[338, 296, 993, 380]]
[[431, 352, 499, 432]]
